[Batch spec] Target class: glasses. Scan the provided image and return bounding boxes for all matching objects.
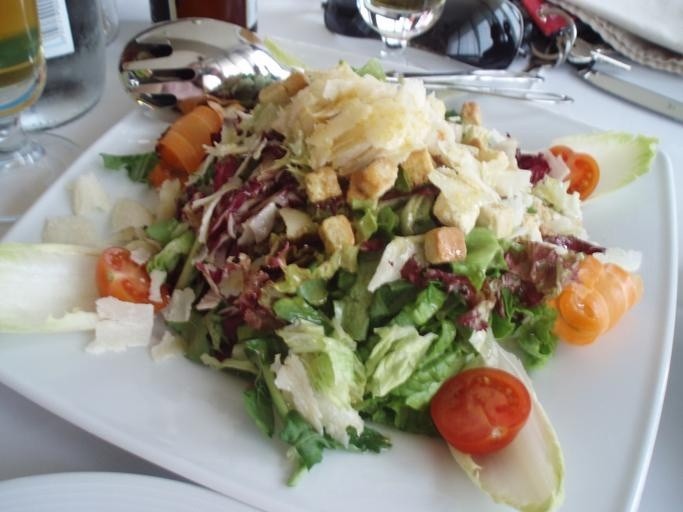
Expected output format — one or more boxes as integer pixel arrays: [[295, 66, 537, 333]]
[[323, 0, 524, 70]]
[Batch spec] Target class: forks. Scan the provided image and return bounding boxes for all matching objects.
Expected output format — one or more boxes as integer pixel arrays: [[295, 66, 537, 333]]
[[117, 17, 576, 124]]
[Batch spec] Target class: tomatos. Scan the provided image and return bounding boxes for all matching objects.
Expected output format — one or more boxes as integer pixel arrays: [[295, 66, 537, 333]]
[[432, 366, 532, 455], [552, 145, 600, 200], [98, 246, 169, 311]]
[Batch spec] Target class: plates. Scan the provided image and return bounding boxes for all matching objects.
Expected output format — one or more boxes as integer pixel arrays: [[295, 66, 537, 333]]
[[0, 33, 681, 511]]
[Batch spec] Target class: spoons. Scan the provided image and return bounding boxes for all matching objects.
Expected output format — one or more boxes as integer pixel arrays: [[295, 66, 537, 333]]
[[120, 15, 547, 108]]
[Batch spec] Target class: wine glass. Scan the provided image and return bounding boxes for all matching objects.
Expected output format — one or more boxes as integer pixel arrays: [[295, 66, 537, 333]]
[[355, 0, 446, 80], [1, 1, 85, 224]]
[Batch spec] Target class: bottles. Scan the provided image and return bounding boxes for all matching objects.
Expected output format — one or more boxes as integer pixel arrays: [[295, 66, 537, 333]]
[[14, 1, 106, 133]]
